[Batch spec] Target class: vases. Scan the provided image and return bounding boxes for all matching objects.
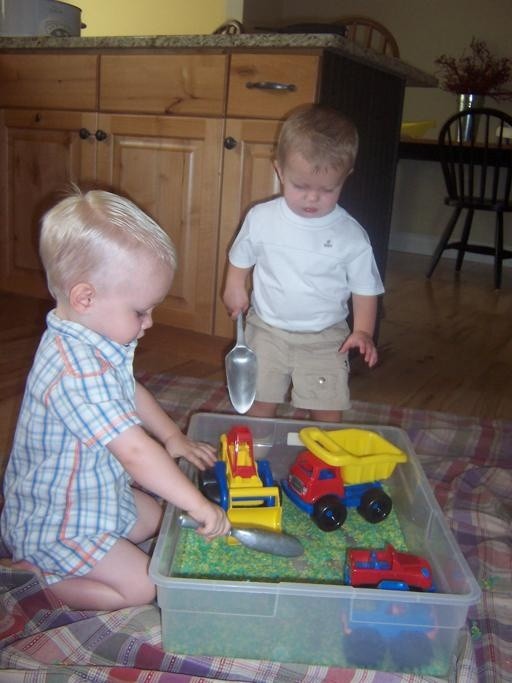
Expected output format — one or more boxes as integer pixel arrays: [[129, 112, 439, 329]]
[[453, 91, 485, 146]]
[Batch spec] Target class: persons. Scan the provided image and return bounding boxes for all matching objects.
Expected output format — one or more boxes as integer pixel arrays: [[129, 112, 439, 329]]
[[0, 189, 233, 611], [221, 101, 386, 458]]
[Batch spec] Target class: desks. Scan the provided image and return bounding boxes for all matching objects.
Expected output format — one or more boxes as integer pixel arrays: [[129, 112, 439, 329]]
[[397, 134, 510, 166]]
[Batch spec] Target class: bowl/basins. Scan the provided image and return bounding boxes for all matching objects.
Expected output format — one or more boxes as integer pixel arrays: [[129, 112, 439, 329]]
[[401, 120, 436, 138]]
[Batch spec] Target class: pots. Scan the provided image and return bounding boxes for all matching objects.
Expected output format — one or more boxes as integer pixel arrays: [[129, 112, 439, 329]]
[[1, 0, 88, 37]]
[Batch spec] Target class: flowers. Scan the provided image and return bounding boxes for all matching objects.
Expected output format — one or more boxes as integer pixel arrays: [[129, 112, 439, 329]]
[[430, 36, 512, 100]]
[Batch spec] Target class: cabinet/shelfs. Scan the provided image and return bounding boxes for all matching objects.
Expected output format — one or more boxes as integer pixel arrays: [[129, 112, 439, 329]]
[[0, 32, 229, 338], [212, 50, 410, 346]]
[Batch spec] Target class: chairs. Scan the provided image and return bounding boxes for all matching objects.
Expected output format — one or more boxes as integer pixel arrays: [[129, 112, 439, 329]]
[[209, 19, 243, 33], [427, 106, 512, 287], [338, 17, 399, 54]]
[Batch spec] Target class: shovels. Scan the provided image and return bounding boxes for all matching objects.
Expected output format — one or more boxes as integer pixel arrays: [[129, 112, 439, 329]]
[[225, 310, 257, 414], [177, 515, 305, 558]]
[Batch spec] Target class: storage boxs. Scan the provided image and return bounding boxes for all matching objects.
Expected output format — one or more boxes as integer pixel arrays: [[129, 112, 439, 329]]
[[146, 408, 482, 678]]
